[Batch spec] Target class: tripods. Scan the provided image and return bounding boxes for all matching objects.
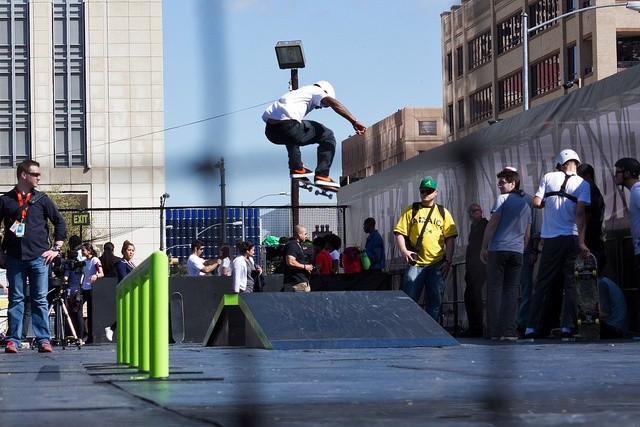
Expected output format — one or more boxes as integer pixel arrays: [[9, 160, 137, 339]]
[[29, 289, 81, 350]]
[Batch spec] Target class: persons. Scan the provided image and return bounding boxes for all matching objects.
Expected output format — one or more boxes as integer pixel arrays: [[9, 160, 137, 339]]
[[81, 242, 104, 344], [99, 242, 122, 277], [480, 167, 543, 340], [591, 251, 626, 339], [0, 160, 67, 353], [105, 240, 137, 341], [233, 242, 262, 293], [393, 178, 458, 321], [460, 204, 491, 338], [283, 225, 313, 292], [312, 237, 332, 275], [218, 246, 232, 276], [363, 218, 385, 272], [517, 149, 605, 341], [614, 157, 640, 339], [187, 240, 222, 276], [323, 234, 341, 274], [262, 80, 367, 188]]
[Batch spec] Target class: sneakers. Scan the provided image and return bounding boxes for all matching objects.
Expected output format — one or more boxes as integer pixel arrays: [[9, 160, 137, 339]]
[[551, 326, 571, 337], [289, 165, 315, 178], [104, 327, 114, 341], [313, 175, 340, 188], [561, 332, 575, 342], [37, 342, 53, 352], [5, 341, 17, 352], [516, 333, 535, 343]]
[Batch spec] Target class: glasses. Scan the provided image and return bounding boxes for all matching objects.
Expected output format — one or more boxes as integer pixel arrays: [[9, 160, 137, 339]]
[[26, 171, 41, 177], [616, 170, 623, 175], [467, 209, 481, 214]]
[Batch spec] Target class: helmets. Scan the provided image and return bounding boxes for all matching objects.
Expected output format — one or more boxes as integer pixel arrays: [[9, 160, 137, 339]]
[[614, 158, 640, 192], [555, 149, 581, 169], [314, 80, 335, 98]]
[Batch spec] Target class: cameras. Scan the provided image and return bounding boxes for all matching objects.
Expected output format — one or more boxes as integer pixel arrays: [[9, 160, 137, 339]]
[[407, 244, 418, 265], [311, 264, 320, 273], [49, 249, 87, 289]]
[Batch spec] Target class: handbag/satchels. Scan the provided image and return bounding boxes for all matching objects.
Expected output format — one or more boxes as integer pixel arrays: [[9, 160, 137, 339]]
[[0, 191, 49, 269]]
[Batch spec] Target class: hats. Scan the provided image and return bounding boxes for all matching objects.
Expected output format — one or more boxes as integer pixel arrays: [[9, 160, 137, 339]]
[[418, 179, 438, 190]]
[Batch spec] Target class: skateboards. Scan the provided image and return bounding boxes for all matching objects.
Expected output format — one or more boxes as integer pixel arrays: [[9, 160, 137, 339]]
[[293, 177, 340, 200], [574, 254, 601, 341]]
